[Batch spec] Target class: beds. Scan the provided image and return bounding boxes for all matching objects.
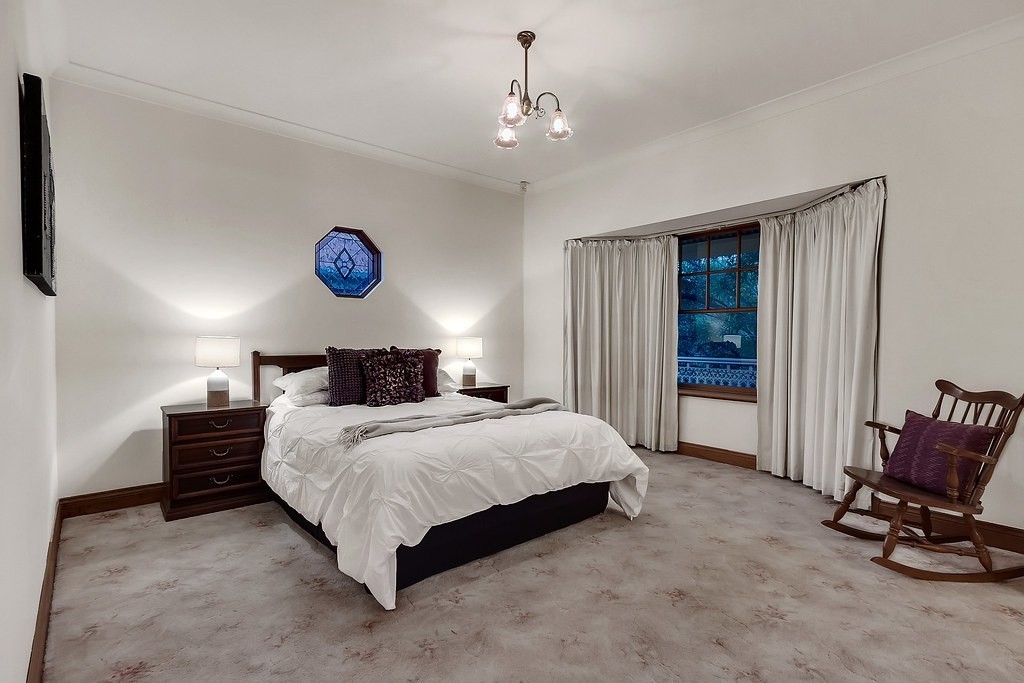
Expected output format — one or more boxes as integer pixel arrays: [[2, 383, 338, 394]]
[[248, 349, 652, 615]]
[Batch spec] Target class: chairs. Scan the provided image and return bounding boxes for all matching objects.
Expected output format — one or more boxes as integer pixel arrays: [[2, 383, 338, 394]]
[[821, 376, 1024, 586]]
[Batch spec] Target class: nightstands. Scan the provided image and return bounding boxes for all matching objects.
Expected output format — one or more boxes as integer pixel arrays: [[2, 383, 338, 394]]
[[453, 379, 512, 405]]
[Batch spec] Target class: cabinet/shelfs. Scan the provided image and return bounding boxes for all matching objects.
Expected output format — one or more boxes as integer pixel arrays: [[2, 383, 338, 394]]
[[160, 398, 273, 519]]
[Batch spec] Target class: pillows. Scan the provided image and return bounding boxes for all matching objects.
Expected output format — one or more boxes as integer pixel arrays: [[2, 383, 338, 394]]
[[434, 368, 460, 395], [270, 364, 329, 408], [322, 345, 444, 408], [358, 350, 426, 407], [882, 407, 1002, 503]]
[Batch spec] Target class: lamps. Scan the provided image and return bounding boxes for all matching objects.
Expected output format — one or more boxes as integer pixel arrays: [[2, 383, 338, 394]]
[[493, 30, 575, 153], [451, 335, 486, 388], [194, 334, 243, 409]]
